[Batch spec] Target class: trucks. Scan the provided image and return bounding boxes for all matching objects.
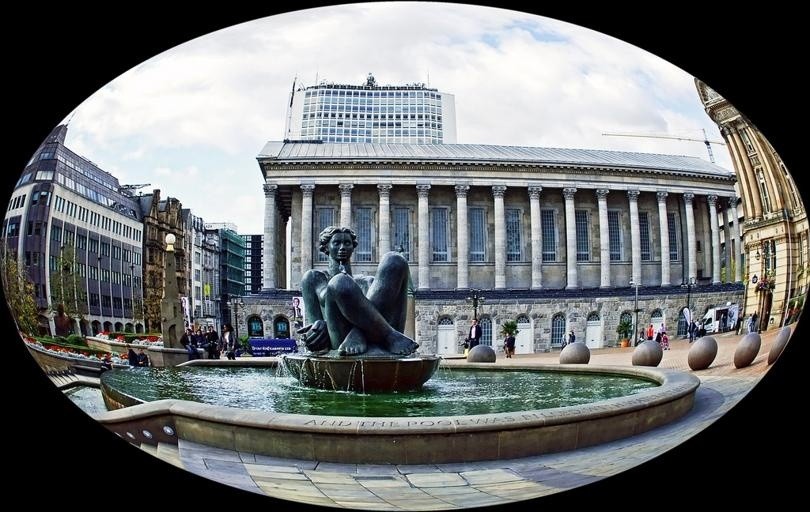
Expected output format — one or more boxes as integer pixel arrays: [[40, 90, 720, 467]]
[[702, 302, 739, 334]]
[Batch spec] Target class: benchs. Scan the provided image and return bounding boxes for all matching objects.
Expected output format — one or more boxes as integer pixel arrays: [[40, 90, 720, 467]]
[[70, 364, 100, 376]]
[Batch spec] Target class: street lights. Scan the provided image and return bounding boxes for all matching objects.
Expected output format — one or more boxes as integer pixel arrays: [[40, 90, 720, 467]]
[[227, 294, 244, 339], [629, 277, 642, 347], [680, 276, 698, 340], [754, 239, 774, 334], [466, 288, 486, 320]]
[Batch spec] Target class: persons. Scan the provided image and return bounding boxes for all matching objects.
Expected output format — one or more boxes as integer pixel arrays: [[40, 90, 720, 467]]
[[662, 334, 670, 350], [561, 339, 567, 349], [137, 349, 148, 367], [568, 334, 572, 344], [507, 334, 515, 358], [656, 333, 661, 345], [695, 319, 701, 337], [503, 333, 509, 357], [647, 325, 654, 340], [179, 322, 236, 361], [688, 320, 697, 343], [468, 319, 482, 349], [700, 320, 704, 337], [720, 310, 758, 333], [571, 332, 575, 342], [103, 352, 112, 371], [659, 324, 664, 334], [293, 298, 302, 318], [297, 226, 420, 356]]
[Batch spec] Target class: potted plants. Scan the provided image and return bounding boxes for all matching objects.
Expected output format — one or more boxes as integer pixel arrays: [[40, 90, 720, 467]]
[[617, 322, 632, 348]]
